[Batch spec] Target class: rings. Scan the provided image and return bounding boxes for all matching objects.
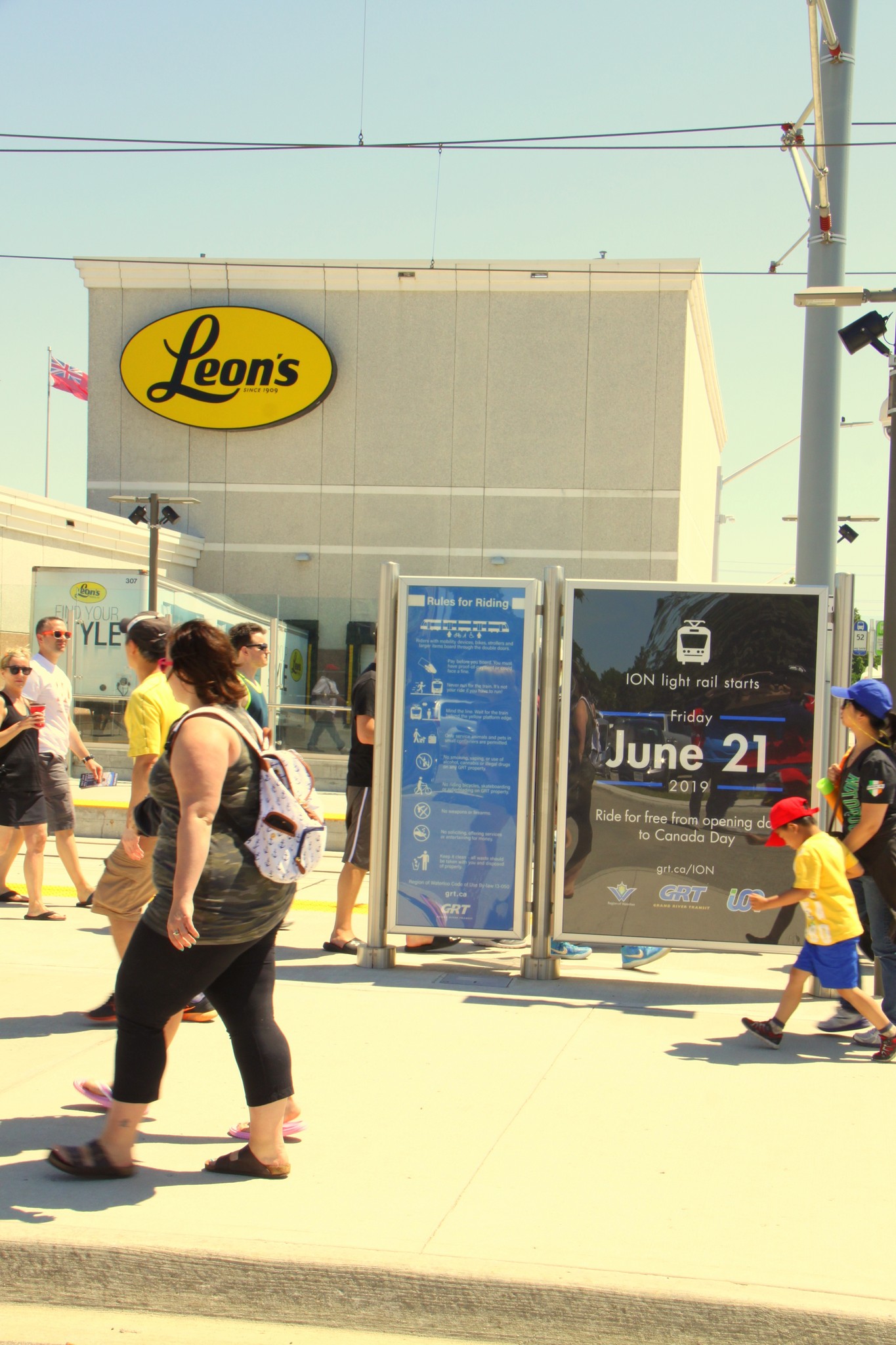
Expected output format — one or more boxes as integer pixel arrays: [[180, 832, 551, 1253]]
[[173, 930, 181, 936]]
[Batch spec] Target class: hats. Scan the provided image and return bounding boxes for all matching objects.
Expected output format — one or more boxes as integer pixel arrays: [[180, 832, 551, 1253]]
[[764, 797, 819, 847], [323, 663, 339, 671], [830, 679, 893, 720], [119, 610, 172, 654], [761, 768, 809, 806]]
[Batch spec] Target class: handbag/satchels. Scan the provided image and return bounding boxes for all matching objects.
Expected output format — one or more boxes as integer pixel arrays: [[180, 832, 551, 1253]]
[[308, 697, 333, 722]]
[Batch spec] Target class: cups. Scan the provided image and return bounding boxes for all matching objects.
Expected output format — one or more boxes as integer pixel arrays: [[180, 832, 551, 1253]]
[[30, 705, 46, 727]]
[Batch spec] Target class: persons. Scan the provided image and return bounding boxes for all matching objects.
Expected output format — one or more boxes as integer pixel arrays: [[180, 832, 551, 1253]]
[[308, 660, 351, 755], [745, 766, 810, 944], [457, 667, 519, 928], [550, 869, 673, 969], [742, 797, 896, 1062], [49, 616, 299, 1179], [564, 658, 598, 899], [816, 677, 896, 1049], [689, 684, 739, 833], [231, 621, 296, 928], [78, 610, 219, 1024], [0, 646, 69, 921], [21, 615, 104, 907], [323, 624, 461, 955]]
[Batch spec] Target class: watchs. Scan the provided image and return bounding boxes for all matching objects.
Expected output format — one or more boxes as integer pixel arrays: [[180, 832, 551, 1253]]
[[82, 754, 95, 763]]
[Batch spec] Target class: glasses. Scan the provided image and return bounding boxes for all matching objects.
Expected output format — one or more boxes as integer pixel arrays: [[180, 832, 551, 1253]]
[[842, 700, 849, 710], [244, 643, 268, 649], [40, 630, 73, 640], [2, 665, 32, 675]]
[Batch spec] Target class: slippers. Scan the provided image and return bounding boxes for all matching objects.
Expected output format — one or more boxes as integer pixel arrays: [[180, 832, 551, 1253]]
[[0, 890, 29, 903], [323, 937, 366, 955], [404, 936, 461, 953], [24, 911, 66, 921], [73, 1077, 149, 1115], [76, 890, 96, 907], [229, 1120, 308, 1139]]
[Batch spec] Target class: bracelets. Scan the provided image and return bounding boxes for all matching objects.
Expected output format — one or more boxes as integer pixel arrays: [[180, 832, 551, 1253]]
[[324, 693, 327, 699]]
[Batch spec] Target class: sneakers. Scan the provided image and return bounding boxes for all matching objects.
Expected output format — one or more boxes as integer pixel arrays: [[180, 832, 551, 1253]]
[[872, 1034, 896, 1062], [742, 1017, 783, 1049], [182, 996, 219, 1022], [620, 944, 672, 970], [85, 992, 117, 1021], [550, 936, 592, 959]]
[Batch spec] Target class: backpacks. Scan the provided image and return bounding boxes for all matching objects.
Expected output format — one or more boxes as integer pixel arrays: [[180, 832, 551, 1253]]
[[572, 696, 606, 770], [163, 706, 328, 884]]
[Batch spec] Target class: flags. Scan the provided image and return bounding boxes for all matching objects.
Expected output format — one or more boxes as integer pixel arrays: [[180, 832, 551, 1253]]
[[50, 355, 88, 401]]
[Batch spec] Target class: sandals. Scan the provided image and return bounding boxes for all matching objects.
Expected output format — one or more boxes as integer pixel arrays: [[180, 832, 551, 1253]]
[[47, 1139, 139, 1178], [204, 1143, 291, 1178]]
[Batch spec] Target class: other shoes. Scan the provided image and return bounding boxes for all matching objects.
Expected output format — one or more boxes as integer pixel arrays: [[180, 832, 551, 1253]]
[[307, 746, 324, 752], [690, 820, 700, 831], [706, 821, 728, 833], [817, 1005, 870, 1032], [339, 746, 351, 755], [471, 938, 527, 949], [853, 1027, 881, 1048], [280, 920, 296, 928]]
